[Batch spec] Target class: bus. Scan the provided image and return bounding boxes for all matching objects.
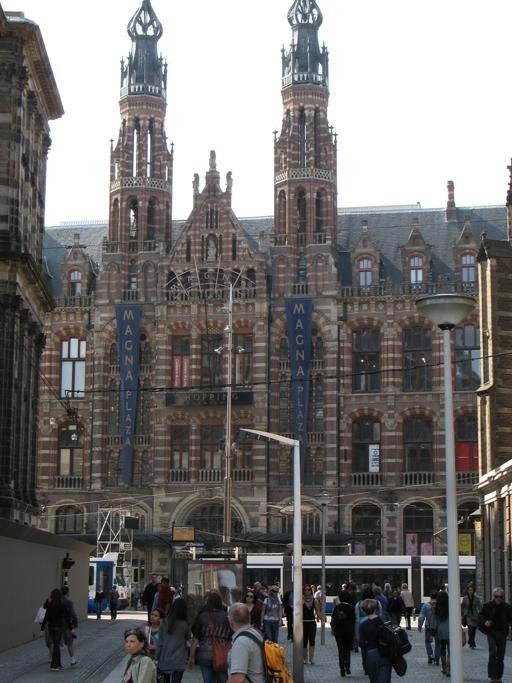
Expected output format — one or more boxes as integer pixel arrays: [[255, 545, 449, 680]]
[[199, 554, 477, 618], [88, 551, 130, 614]]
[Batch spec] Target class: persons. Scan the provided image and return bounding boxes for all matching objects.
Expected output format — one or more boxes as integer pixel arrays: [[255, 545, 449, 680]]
[[330, 590, 354, 676], [38, 587, 78, 671], [359, 599, 391, 682], [301, 584, 322, 664], [481, 587, 512, 682], [355, 588, 383, 675], [95, 584, 119, 620], [122, 574, 295, 683], [341, 583, 414, 651], [461, 586, 481, 648], [418, 584, 450, 677]]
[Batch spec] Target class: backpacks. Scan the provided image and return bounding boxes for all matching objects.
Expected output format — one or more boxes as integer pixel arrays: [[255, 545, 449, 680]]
[[213, 641, 231, 671], [377, 617, 411, 656], [234, 631, 294, 683]]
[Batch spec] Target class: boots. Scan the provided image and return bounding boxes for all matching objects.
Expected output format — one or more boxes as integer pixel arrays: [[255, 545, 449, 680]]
[[429, 655, 450, 676], [309, 646, 314, 663], [303, 648, 308, 664]]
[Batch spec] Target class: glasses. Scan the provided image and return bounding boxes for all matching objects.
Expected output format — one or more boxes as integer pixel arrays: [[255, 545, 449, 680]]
[[495, 596, 503, 599]]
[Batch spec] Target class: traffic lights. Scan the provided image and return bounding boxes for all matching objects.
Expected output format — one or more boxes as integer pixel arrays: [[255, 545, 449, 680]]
[[63, 557, 76, 569]]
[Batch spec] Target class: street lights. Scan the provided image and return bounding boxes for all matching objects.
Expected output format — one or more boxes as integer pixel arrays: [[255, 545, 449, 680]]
[[239, 425, 304, 682], [279, 489, 333, 645], [213, 282, 245, 559], [414, 292, 477, 682]]
